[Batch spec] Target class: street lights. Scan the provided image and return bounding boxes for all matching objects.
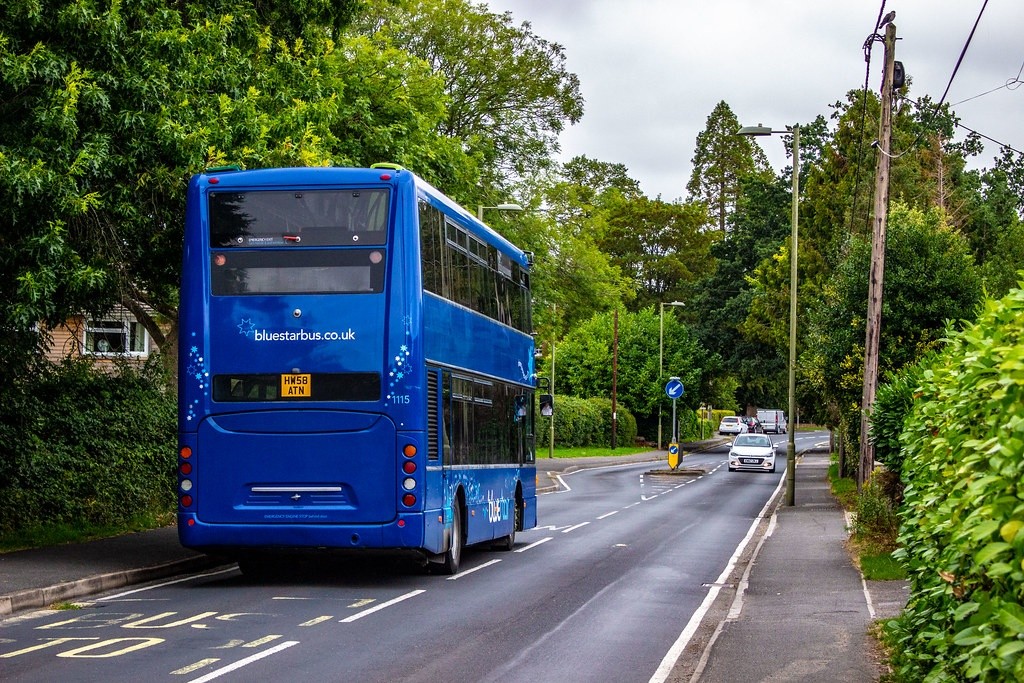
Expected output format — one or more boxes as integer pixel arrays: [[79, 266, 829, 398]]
[[735, 124, 803, 507], [477, 201, 524, 222], [658, 300, 685, 449]]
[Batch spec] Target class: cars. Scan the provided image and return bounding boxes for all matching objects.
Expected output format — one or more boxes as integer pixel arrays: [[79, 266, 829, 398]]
[[718, 416, 750, 435], [725, 433, 779, 473], [745, 416, 764, 433]]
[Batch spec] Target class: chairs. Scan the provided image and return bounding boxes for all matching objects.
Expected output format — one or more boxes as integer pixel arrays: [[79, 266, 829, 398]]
[[759, 439, 766, 446]]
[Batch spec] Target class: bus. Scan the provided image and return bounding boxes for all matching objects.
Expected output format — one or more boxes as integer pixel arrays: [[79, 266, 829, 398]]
[[173, 162, 555, 578]]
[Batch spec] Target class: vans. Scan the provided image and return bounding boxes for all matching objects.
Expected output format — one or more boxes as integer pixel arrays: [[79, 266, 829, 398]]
[[756, 409, 781, 434], [777, 410, 788, 434]]
